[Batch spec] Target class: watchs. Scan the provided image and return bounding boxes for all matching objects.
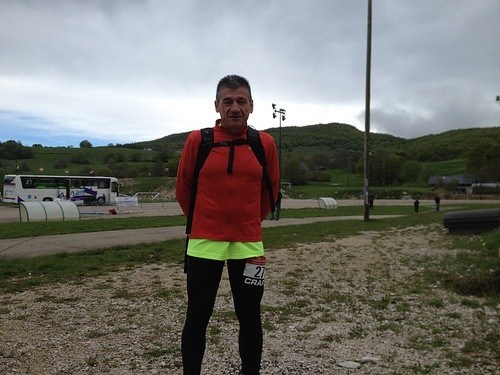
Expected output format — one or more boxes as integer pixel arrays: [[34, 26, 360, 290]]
[[414, 198, 420, 212]]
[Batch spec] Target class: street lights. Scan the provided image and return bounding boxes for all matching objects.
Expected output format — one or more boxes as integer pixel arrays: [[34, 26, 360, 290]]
[[272, 103, 286, 191]]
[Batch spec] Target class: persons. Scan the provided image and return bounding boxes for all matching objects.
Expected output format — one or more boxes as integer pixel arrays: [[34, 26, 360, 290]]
[[369, 192, 374, 209], [435, 193, 440, 211], [177, 75, 280, 375], [270, 192, 282, 221]]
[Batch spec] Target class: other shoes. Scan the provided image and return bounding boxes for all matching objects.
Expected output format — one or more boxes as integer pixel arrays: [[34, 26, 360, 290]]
[[276, 218, 279, 220], [269, 218, 275, 220]]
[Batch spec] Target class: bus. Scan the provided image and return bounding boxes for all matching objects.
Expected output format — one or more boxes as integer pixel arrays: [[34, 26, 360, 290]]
[[1, 174, 122, 205]]
